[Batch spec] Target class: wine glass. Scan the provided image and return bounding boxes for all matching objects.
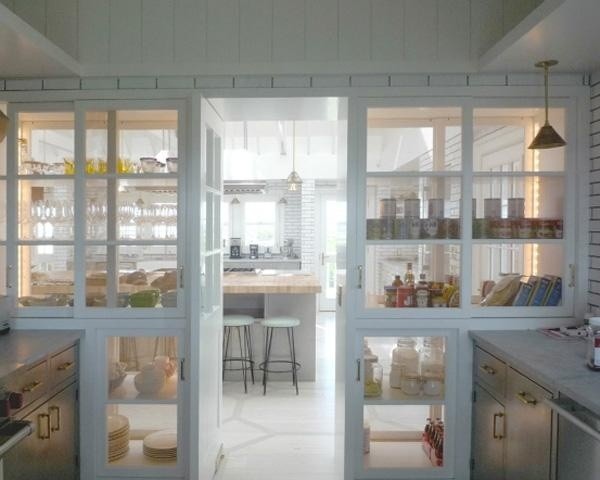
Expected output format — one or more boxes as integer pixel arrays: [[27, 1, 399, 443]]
[[20, 201, 178, 241]]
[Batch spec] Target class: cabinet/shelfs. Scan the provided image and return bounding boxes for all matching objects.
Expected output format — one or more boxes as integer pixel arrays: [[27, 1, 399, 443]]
[[0, 86, 322, 331], [79, 311, 223, 480], [337, 92, 587, 330], [0, 339, 79, 480], [333, 309, 471, 480], [507, 365, 553, 480], [470, 342, 506, 480]]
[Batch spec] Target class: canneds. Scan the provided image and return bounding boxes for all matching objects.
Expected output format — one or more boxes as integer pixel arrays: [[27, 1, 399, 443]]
[[472, 198, 562, 239], [367, 199, 458, 240]]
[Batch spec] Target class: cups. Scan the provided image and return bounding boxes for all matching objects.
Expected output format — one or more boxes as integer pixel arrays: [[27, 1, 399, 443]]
[[21, 156, 178, 174]]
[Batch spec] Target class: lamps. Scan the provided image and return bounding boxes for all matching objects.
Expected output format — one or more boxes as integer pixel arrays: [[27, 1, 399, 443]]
[[527, 59, 567, 150]]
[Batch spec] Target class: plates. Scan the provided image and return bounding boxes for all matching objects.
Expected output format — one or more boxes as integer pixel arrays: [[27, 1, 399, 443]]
[[108, 413, 129, 462], [143, 429, 177, 459]]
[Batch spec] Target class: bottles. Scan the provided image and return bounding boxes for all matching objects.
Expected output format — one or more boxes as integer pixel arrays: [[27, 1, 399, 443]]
[[423, 417, 443, 465], [405, 263, 414, 286], [415, 273, 430, 307], [393, 276, 402, 286], [365, 336, 443, 397]]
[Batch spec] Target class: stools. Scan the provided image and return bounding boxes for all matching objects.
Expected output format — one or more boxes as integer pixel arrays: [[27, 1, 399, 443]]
[[222, 314, 255, 393], [259, 317, 302, 395]]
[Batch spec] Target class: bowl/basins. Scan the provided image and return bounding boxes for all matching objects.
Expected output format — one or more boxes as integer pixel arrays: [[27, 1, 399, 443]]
[[17, 287, 176, 307]]
